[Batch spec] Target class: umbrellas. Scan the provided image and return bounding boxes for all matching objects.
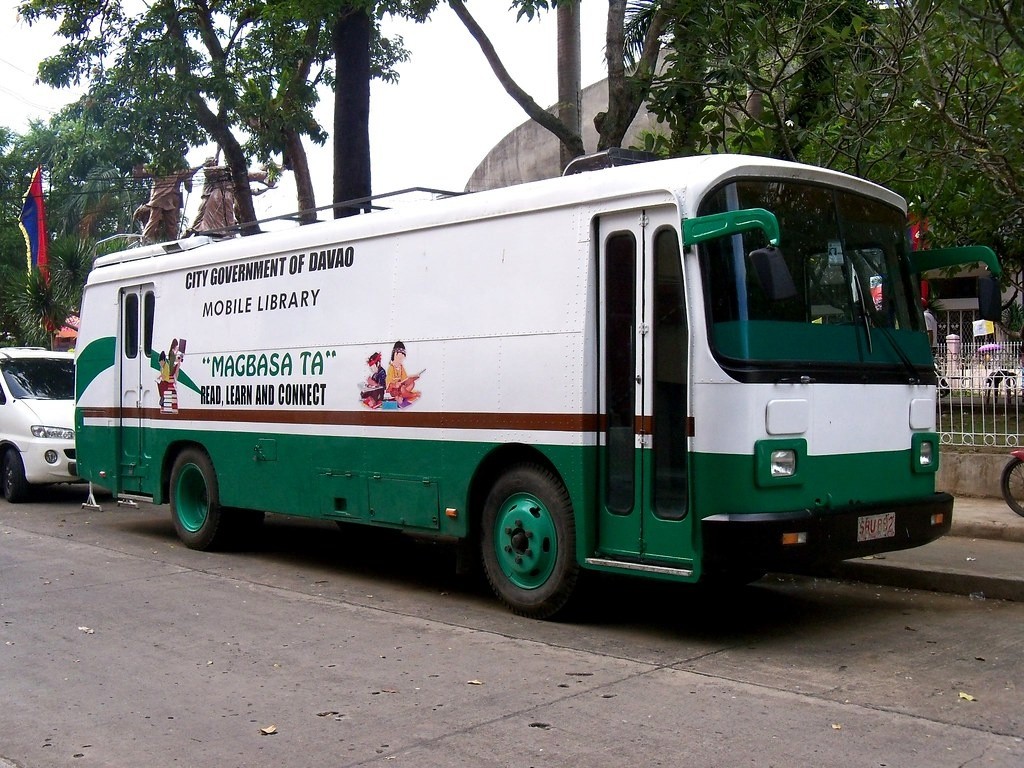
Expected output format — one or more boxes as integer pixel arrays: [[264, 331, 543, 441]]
[[978, 344, 1004, 352]]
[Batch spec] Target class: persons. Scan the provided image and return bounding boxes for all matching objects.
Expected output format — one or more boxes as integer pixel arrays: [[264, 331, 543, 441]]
[[134, 148, 271, 246], [920, 298, 952, 398]]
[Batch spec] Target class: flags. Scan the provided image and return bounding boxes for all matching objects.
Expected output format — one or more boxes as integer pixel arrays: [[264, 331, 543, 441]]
[[16, 168, 51, 294]]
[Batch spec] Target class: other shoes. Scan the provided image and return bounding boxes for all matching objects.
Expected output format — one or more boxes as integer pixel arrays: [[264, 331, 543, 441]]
[[937, 387, 953, 398]]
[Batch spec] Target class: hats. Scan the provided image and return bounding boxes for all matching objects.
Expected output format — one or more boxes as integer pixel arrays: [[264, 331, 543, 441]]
[[921, 298, 928, 306]]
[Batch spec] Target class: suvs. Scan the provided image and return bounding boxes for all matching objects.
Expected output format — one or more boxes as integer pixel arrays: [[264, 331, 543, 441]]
[[0, 347, 82, 503]]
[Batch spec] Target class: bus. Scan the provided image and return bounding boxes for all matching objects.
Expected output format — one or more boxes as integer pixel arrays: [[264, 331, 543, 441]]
[[74, 146, 1006, 617]]
